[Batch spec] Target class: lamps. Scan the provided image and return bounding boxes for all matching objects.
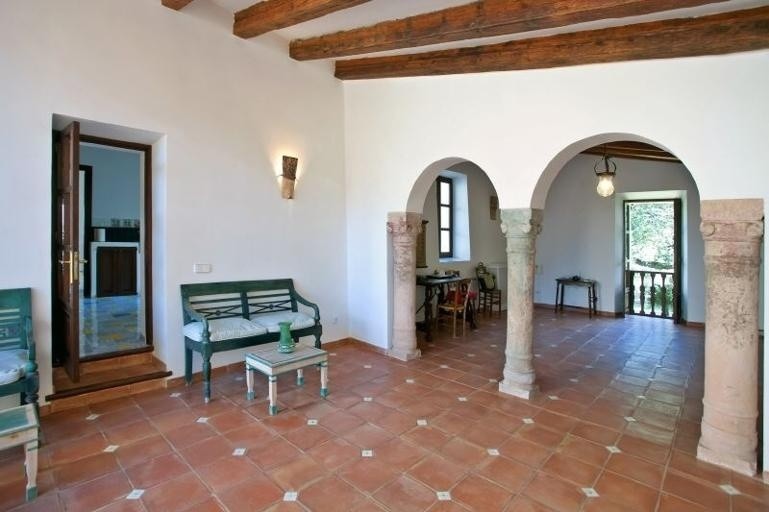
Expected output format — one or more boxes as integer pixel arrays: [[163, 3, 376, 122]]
[[282, 156, 298, 199], [594, 150, 617, 198]]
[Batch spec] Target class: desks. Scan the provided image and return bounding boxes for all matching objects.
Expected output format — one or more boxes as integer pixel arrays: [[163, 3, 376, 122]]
[[417, 274, 476, 342], [553, 275, 597, 319]]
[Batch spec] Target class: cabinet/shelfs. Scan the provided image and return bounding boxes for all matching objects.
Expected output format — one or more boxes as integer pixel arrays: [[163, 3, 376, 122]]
[[94, 237, 138, 296]]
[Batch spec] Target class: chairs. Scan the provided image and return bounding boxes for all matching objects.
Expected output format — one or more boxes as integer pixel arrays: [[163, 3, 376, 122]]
[[477, 262, 502, 315], [435, 277, 474, 340]]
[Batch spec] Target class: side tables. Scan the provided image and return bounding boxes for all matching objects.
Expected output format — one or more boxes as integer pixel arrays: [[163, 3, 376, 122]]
[[246, 343, 331, 416], [0, 402, 40, 503]]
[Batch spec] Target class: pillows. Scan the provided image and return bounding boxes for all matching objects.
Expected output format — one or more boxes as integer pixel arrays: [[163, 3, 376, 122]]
[[477, 263, 496, 290]]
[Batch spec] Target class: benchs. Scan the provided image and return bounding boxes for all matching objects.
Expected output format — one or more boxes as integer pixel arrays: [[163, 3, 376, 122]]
[[0, 288, 43, 445], [180, 279, 321, 402]]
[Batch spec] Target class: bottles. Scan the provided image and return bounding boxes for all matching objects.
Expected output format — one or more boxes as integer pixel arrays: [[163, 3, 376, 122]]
[[278, 323, 295, 354]]
